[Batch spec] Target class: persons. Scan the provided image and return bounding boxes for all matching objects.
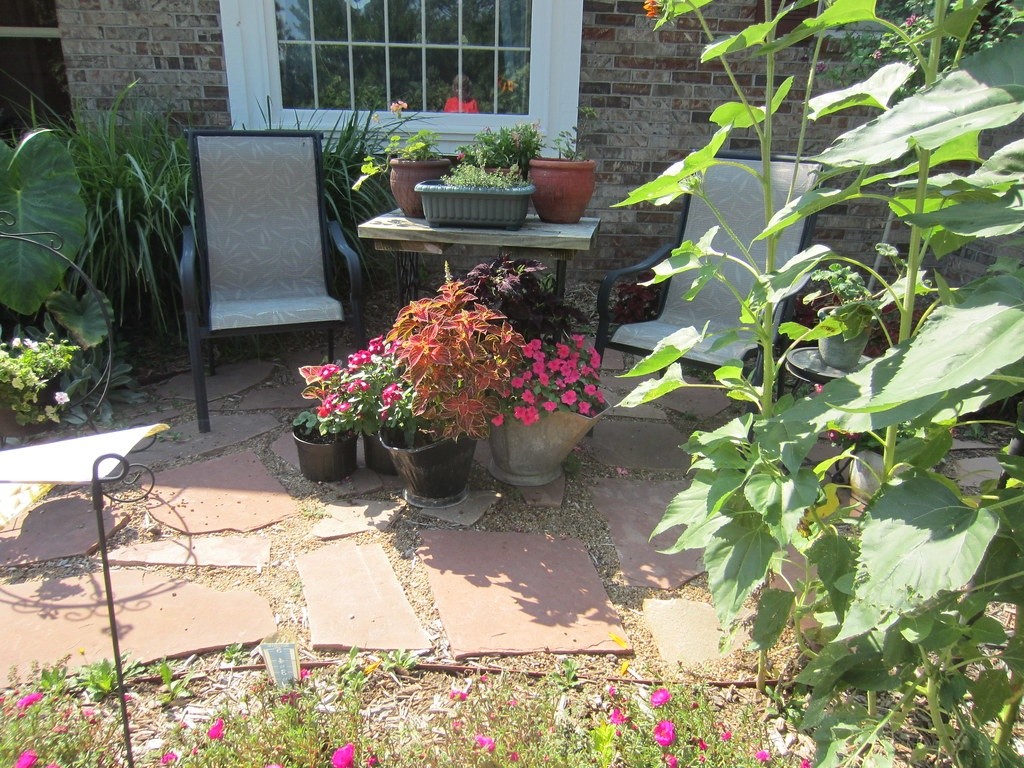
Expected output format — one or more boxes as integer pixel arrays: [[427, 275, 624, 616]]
[[443, 72, 480, 113]]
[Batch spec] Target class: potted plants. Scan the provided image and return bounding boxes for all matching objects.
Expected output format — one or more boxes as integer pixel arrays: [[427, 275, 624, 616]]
[[801, 243, 900, 371], [412, 162, 536, 231], [530, 105, 599, 223]]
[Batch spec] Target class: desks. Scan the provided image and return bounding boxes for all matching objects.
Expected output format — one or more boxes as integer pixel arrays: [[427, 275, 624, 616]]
[[784, 348, 872, 485], [358, 208, 601, 345]]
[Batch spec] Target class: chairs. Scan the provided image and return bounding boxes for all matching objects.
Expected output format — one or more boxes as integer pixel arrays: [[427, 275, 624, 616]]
[[176, 129, 366, 433], [584, 149, 827, 476]]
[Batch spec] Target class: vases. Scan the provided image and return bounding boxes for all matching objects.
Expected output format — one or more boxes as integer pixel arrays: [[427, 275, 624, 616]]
[[487, 397, 612, 486], [361, 429, 399, 476], [388, 157, 451, 219], [377, 423, 478, 510], [292, 429, 359, 483], [477, 165, 522, 176], [0, 408, 51, 437]]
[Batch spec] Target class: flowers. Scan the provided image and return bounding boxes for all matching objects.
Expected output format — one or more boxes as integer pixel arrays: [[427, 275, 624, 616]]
[[293, 251, 614, 444], [0, 331, 82, 425], [348, 90, 442, 191], [455, 122, 546, 167]]
